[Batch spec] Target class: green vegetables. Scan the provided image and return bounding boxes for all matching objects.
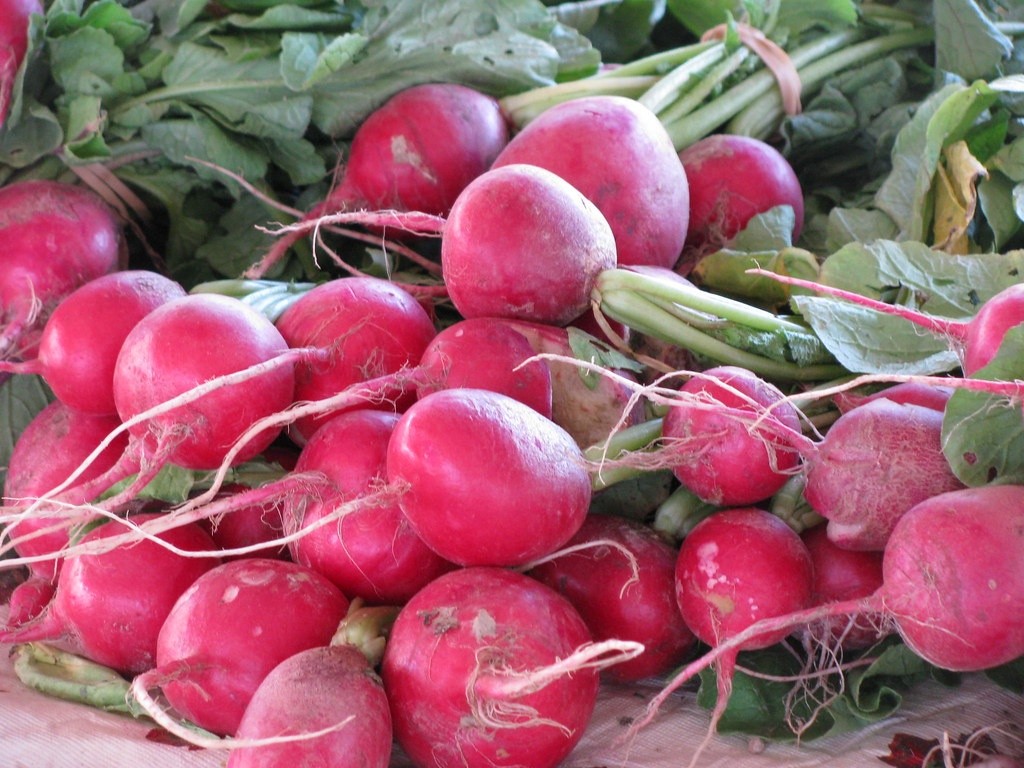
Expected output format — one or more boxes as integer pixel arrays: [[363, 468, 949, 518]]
[[1, 0, 1024, 487]]
